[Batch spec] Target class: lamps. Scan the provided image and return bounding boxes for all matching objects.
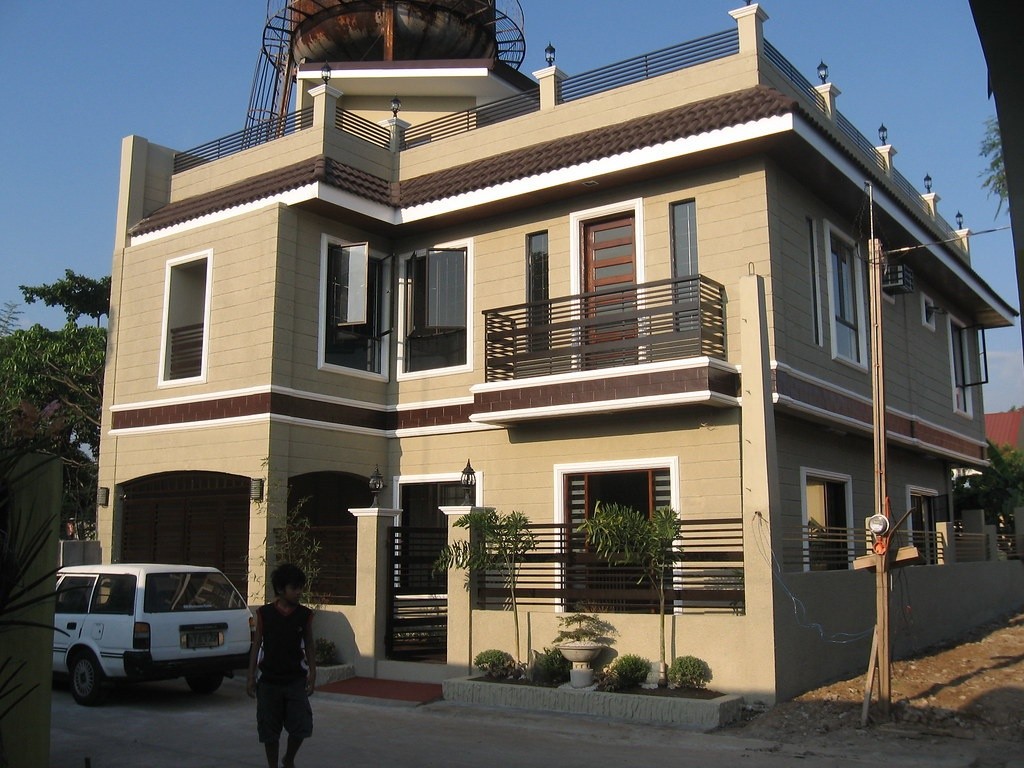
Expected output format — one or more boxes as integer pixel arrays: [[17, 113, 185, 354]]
[[97, 487, 109, 507], [369, 463, 384, 507], [956, 210, 964, 230], [320, 60, 331, 85], [924, 172, 932, 194], [545, 41, 555, 66], [817, 58, 829, 84], [877, 122, 887, 146], [251, 478, 263, 501], [460, 459, 476, 506], [389, 92, 401, 118]]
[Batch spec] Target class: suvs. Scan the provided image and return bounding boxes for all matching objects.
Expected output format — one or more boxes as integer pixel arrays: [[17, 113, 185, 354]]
[[53, 563, 257, 705]]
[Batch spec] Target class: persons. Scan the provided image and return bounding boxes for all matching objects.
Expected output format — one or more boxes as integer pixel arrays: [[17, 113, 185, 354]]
[[245, 562, 317, 768]]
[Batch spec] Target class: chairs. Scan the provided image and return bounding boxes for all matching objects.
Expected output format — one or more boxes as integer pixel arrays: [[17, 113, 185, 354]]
[[65, 591, 88, 613]]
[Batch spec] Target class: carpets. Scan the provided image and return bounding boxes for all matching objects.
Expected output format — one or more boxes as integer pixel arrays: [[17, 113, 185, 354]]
[[313, 676, 442, 702]]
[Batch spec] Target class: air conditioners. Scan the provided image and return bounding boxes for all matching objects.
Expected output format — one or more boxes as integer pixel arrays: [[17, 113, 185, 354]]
[[888, 266, 913, 292]]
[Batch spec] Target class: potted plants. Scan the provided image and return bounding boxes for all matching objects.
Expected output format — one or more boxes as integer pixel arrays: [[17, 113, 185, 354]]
[[551, 615, 602, 661]]
